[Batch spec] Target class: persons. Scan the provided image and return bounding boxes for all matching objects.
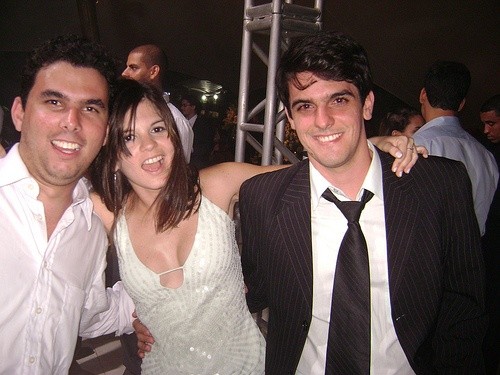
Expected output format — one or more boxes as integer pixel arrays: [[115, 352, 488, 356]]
[[0, 32, 140, 375], [70, 44, 238, 375], [0, 76, 431, 375], [476, 94, 500, 163], [411, 59, 500, 240], [377, 106, 427, 142], [131, 30, 489, 375]]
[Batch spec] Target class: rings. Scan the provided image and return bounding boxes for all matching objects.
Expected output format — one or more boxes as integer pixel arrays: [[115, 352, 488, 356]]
[[407, 147, 413, 151]]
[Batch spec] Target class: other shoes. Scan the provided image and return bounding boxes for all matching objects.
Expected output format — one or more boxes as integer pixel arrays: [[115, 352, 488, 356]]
[[75, 347, 97, 375]]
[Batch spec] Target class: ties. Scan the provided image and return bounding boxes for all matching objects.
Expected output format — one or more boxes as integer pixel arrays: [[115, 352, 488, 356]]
[[321, 187, 375, 375]]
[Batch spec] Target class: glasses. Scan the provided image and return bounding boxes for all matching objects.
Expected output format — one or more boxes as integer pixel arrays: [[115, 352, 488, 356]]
[[181, 103, 192, 107]]
